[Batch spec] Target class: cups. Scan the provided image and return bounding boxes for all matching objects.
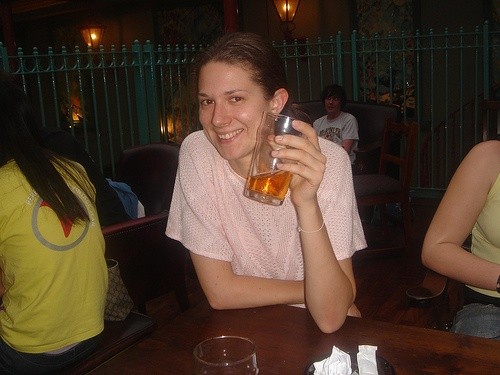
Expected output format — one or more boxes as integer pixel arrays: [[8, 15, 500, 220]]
[[243, 111, 310, 206], [192, 335, 259, 375]]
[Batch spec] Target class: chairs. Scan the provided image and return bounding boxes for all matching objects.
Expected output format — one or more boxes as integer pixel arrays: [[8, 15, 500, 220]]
[[357, 119, 420, 247], [120, 143, 181, 215]]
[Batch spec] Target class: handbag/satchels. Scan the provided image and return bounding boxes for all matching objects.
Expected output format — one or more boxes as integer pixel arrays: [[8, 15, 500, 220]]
[[104, 259, 135, 322]]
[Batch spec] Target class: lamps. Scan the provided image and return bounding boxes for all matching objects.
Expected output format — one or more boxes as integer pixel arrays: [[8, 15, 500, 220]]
[[80, 5, 106, 49], [273, 0, 302, 38]]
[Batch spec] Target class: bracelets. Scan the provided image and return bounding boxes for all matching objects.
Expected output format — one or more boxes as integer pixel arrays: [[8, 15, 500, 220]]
[[297, 221, 324, 234], [496, 275, 500, 294]]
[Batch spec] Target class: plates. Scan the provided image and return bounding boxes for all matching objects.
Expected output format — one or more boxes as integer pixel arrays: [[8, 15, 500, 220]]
[[304, 351, 395, 375]]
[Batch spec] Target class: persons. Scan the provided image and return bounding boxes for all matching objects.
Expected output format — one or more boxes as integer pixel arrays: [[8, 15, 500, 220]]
[[165, 31, 367, 333], [422, 141, 500, 340], [0, 78, 148, 375], [313, 84, 359, 167]]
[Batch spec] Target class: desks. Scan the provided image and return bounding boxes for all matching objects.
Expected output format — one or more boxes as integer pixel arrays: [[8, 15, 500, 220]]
[[85, 303, 500, 375]]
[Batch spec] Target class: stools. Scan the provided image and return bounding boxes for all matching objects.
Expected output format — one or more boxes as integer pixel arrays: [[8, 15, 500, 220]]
[[66, 309, 157, 375]]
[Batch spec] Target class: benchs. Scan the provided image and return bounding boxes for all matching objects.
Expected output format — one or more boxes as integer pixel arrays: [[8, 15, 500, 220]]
[[103, 210, 192, 312], [293, 100, 401, 175]]
